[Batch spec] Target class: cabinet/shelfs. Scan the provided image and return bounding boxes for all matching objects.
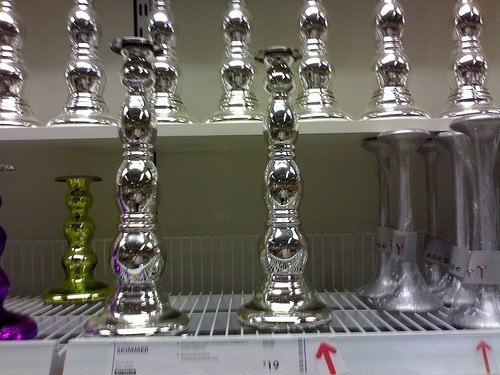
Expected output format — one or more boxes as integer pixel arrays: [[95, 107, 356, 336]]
[[0, 1, 500, 375]]
